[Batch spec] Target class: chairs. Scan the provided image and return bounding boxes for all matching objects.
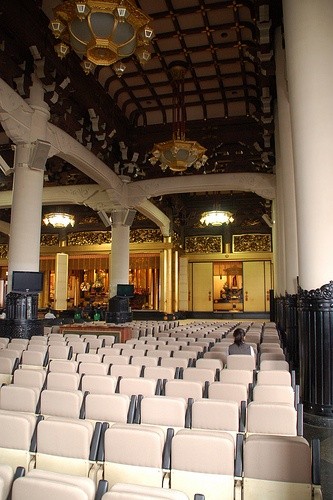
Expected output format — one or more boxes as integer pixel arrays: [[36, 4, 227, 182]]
[[0, 314, 325, 500]]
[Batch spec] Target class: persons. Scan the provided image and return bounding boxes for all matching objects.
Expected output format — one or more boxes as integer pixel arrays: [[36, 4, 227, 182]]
[[229, 329, 253, 356], [44, 308, 56, 320], [0, 309, 7, 320]]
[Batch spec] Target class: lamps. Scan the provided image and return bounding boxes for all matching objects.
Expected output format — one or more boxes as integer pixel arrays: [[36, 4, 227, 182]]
[[198, 191, 234, 227], [148, 63, 211, 173], [42, 206, 76, 229], [49, 2, 156, 78]]
